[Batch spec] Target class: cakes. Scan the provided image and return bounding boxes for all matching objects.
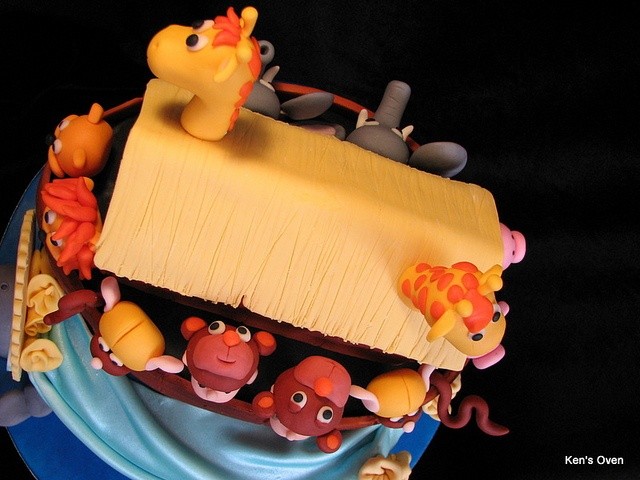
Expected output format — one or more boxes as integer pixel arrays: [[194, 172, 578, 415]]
[[7, 6, 526, 479]]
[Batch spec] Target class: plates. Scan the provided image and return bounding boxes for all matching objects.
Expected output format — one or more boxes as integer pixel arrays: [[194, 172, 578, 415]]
[[0, 157, 444, 480]]
